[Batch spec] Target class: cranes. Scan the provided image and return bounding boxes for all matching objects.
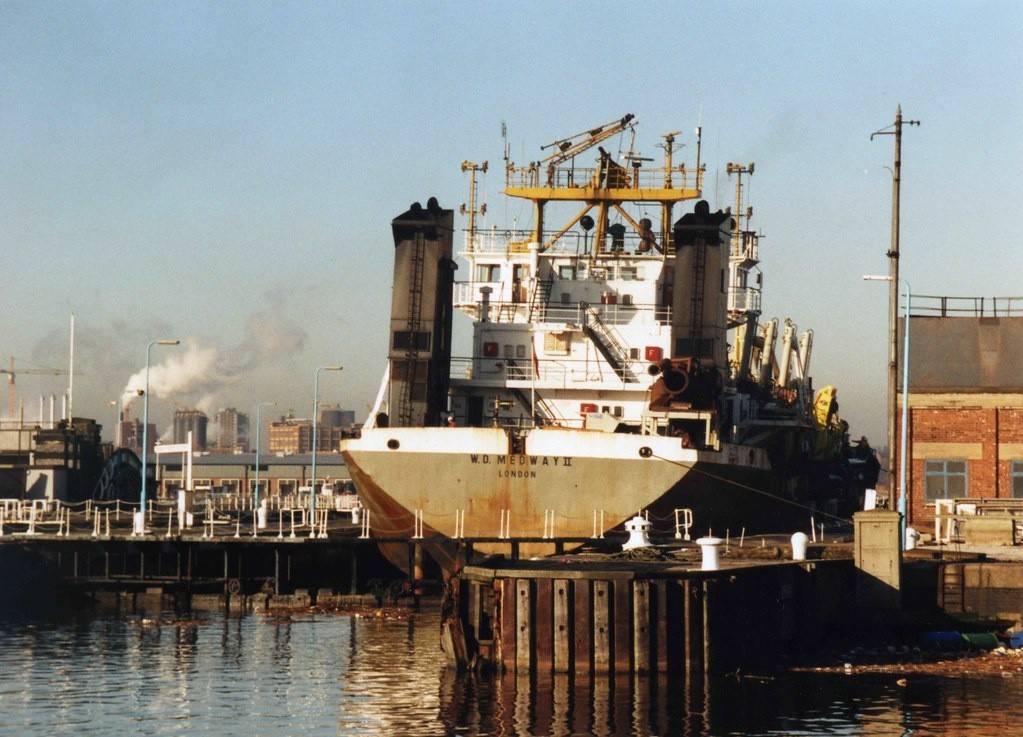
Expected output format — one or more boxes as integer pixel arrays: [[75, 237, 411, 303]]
[[0, 354, 87, 419]]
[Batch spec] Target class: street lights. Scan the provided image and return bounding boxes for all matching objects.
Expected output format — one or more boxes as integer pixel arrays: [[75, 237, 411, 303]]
[[860, 274, 912, 553], [117, 389, 145, 451], [255, 400, 278, 511], [140, 339, 181, 513], [309, 366, 344, 524]]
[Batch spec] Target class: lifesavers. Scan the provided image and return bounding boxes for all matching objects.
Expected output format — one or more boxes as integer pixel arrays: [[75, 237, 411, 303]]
[[672, 429, 691, 448], [390, 580, 406, 597], [367, 578, 385, 593]]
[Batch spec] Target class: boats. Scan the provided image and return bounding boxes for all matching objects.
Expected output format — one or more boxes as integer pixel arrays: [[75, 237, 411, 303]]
[[331, 108, 885, 601]]
